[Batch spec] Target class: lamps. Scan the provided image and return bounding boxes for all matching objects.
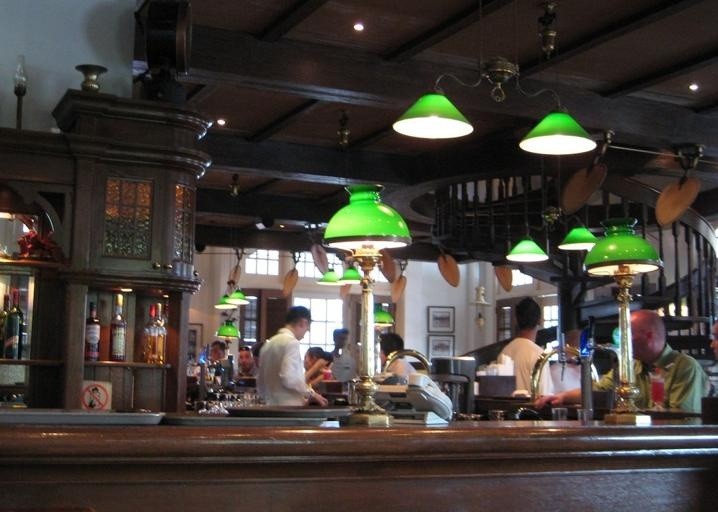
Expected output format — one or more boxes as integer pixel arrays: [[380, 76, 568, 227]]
[[214, 318, 241, 341], [215, 280, 251, 310], [374, 309, 394, 328], [391, 55, 598, 156], [320, 181, 412, 428], [313, 261, 361, 286], [503, 206, 599, 262], [581, 215, 663, 424], [469, 283, 492, 330]]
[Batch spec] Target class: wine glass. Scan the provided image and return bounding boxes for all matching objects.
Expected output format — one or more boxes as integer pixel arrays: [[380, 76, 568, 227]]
[[651, 376, 665, 411]]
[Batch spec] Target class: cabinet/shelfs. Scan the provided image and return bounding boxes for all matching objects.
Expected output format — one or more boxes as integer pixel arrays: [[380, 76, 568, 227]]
[[1, 88, 214, 414]]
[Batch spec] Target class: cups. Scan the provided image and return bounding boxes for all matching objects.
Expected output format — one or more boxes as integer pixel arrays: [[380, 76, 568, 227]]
[[576, 408, 594, 426], [551, 407, 569, 421], [322, 369, 333, 380]]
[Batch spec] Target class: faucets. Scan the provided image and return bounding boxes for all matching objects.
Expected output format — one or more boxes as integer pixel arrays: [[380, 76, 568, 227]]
[[530, 340, 601, 411], [382, 349, 432, 378]]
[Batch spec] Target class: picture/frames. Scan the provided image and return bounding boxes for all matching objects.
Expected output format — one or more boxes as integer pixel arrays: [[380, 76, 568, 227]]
[[427, 335, 456, 363], [188, 322, 202, 365], [428, 305, 455, 333]]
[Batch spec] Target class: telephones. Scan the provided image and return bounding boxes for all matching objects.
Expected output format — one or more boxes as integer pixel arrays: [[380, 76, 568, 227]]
[[370, 373, 454, 417]]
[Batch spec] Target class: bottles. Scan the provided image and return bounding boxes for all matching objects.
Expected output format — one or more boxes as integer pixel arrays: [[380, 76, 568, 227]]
[[0, 294, 12, 360], [6, 289, 23, 360], [84, 300, 101, 361], [141, 302, 167, 365], [108, 288, 128, 361], [205, 355, 239, 393]]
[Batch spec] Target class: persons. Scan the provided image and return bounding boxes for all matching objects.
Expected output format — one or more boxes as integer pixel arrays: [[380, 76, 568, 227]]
[[210, 340, 266, 384], [256, 305, 328, 408], [495, 298, 554, 396], [303, 328, 351, 387], [535, 310, 707, 413], [379, 332, 416, 383], [550, 330, 599, 395]]
